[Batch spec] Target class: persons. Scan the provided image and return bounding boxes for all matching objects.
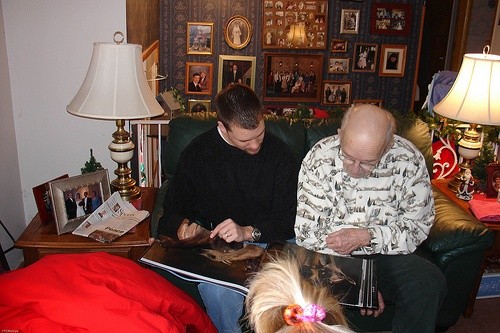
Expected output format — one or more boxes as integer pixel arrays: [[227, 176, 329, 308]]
[[268, 69, 316, 95], [66, 190, 101, 220], [226, 63, 242, 86], [294, 102, 447, 333], [157, 83, 299, 333], [99, 209, 106, 218], [193, 29, 207, 51], [198, 69, 210, 90], [113, 204, 119, 211], [376, 10, 389, 30], [357, 48, 367, 68], [189, 73, 202, 92], [231, 22, 241, 44], [325, 86, 346, 103], [330, 61, 344, 71], [245, 253, 355, 333], [456, 168, 475, 201], [84, 222, 90, 228], [264, 0, 325, 48]]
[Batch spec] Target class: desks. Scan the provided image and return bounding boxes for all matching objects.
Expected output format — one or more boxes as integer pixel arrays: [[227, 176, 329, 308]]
[[13, 185, 159, 270]]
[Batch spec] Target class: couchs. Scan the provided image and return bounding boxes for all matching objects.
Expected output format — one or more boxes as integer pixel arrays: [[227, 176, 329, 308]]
[[147, 113, 493, 333]]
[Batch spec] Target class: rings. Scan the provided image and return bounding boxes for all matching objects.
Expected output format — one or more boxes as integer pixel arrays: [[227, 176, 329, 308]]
[[225, 233, 228, 237]]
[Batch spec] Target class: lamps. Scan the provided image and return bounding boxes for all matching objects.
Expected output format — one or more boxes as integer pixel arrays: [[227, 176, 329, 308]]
[[430, 45, 500, 195], [63, 31, 166, 204]]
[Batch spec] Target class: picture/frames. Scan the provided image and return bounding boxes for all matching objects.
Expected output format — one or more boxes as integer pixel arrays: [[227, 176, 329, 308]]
[[31, 168, 112, 237], [321, 0, 414, 112], [261, 51, 325, 105], [181, 14, 261, 114]]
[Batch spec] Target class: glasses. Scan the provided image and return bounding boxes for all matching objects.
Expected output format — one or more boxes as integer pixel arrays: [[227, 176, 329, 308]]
[[337, 146, 382, 172]]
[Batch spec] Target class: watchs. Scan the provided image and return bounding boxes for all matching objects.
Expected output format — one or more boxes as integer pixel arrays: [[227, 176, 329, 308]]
[[251, 228, 262, 242]]
